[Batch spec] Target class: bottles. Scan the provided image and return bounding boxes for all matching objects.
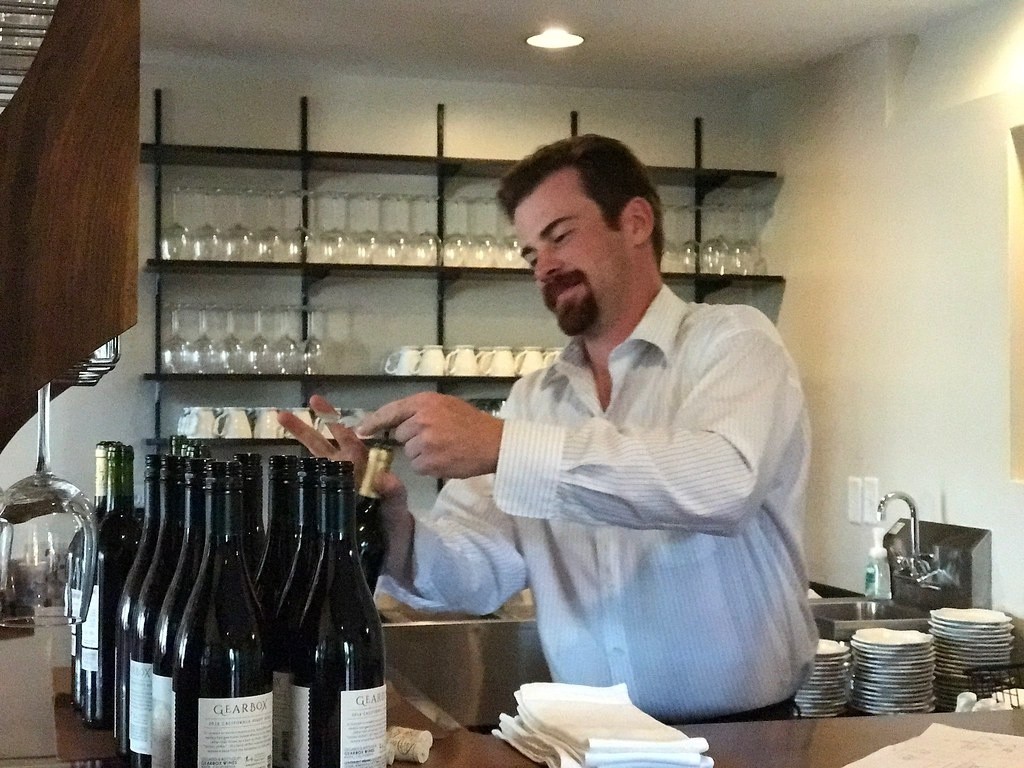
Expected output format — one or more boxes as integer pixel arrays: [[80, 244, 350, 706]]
[[75, 432, 395, 768], [865, 548, 893, 600]]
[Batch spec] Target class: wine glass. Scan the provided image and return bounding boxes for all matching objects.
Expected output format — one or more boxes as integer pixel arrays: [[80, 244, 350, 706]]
[[160, 301, 326, 375], [660, 203, 761, 275], [161, 183, 533, 268], [1, 382, 96, 623]]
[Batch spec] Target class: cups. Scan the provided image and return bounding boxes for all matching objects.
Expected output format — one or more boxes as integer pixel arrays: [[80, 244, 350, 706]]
[[384, 345, 564, 376], [178, 407, 342, 440]]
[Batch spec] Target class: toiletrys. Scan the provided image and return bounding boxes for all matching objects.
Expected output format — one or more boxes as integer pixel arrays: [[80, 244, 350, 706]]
[[865, 526, 892, 599]]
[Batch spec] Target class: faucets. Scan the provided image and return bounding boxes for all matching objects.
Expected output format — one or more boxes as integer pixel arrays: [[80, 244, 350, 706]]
[[876, 492, 934, 577]]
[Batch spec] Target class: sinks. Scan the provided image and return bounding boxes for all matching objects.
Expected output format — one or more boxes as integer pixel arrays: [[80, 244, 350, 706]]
[[808, 597, 931, 642]]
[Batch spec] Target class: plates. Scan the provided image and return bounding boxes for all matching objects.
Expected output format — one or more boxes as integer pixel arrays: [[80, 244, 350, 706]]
[[792, 606, 1015, 719]]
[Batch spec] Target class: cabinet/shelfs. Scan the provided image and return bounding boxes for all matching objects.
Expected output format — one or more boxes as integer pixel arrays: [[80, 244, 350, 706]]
[[136, 144, 784, 445]]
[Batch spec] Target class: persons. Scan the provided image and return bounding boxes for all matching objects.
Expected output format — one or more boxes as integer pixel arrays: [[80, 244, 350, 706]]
[[278, 133, 819, 726]]
